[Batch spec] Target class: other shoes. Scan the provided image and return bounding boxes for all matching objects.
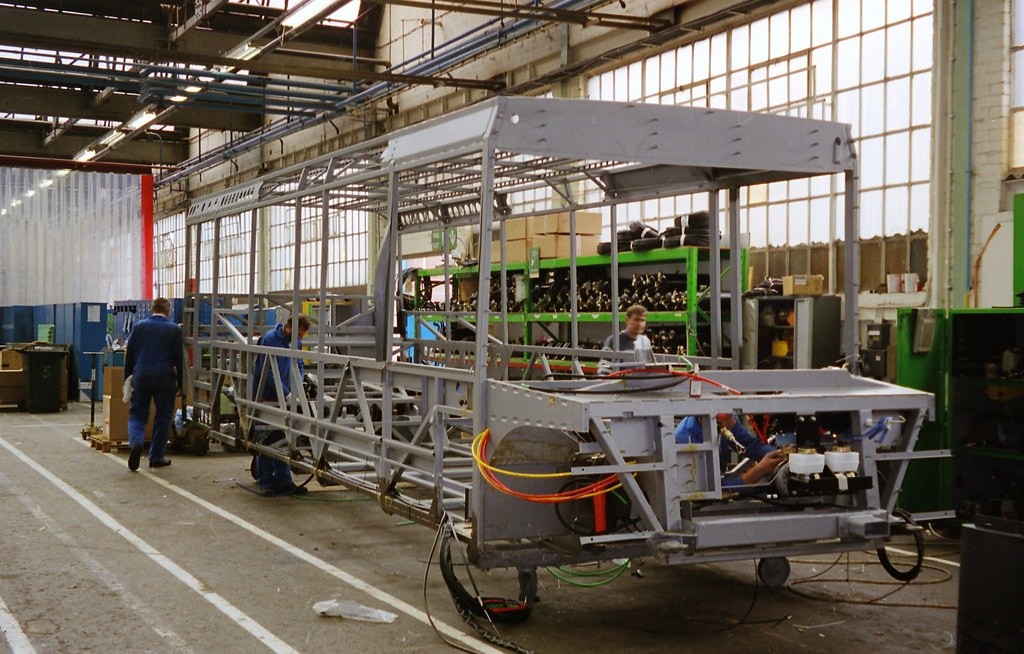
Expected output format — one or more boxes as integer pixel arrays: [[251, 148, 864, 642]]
[[128, 444, 140, 471], [275, 482, 308, 495], [149, 459, 171, 467], [261, 487, 273, 495]]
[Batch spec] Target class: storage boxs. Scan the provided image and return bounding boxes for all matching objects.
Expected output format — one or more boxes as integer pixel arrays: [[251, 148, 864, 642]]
[[102, 394, 156, 441], [781, 274, 824, 296], [103, 366, 127, 398], [465, 210, 604, 262]]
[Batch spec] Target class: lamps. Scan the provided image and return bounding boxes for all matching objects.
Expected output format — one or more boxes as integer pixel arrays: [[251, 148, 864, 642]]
[[73, 108, 157, 161], [206, 39, 259, 74]]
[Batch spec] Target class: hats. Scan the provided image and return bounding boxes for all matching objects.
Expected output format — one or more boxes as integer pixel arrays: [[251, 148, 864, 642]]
[[717, 413, 736, 429]]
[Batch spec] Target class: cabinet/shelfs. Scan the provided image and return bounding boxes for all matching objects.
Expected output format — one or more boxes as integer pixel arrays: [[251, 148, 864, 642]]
[[414, 245, 749, 377], [744, 296, 841, 370], [949, 308, 1024, 519]]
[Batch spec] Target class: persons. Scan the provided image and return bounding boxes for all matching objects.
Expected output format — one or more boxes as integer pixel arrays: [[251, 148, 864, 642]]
[[674, 413, 787, 496], [123, 299, 184, 471], [597, 305, 656, 375], [251, 316, 309, 495]]
